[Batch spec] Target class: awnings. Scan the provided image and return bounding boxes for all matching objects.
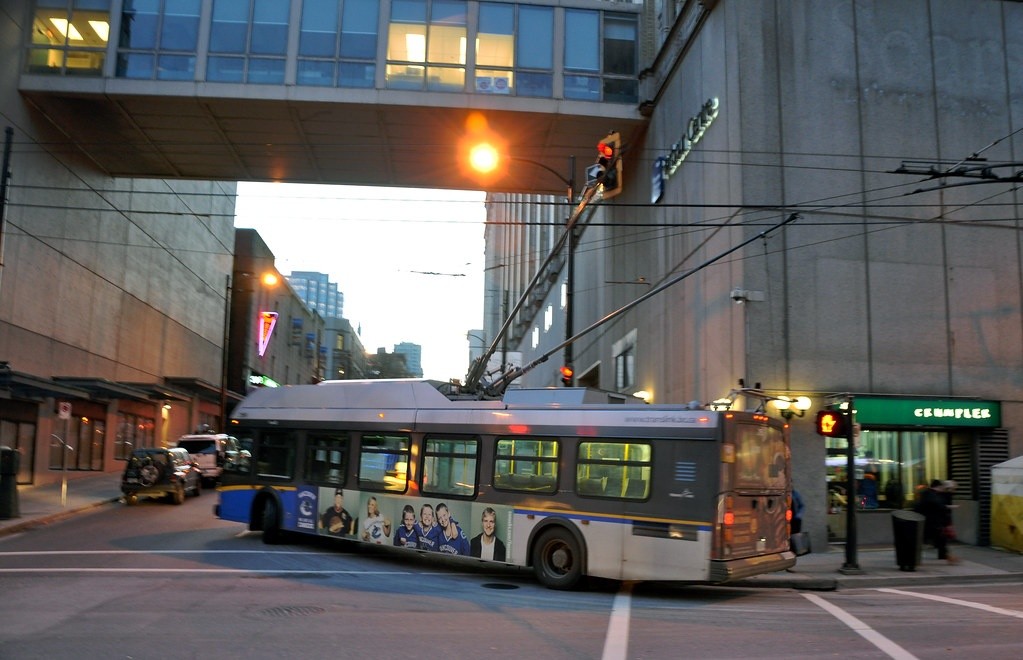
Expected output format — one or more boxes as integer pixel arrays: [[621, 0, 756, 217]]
[[164, 375, 246, 408], [116, 379, 195, 404], [0, 367, 90, 401], [48, 374, 160, 406]]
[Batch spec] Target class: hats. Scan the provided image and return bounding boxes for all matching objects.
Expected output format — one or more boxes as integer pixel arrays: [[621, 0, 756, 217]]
[[942, 480, 957, 489], [930, 480, 944, 486], [335, 488, 343, 496]]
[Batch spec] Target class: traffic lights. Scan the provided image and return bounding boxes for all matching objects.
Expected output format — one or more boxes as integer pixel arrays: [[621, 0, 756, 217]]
[[559, 366, 575, 387], [814, 410, 848, 438], [596, 133, 624, 200]]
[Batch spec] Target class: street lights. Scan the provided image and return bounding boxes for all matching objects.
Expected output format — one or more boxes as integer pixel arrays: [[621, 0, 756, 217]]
[[219, 270, 279, 433], [467, 142, 579, 387]]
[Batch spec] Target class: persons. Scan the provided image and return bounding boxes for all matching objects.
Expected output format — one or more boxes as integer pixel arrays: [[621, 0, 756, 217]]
[[319, 488, 354, 537], [471, 506, 506, 562], [361, 495, 391, 545], [393, 502, 469, 556], [790, 478, 805, 556], [826, 460, 957, 563]]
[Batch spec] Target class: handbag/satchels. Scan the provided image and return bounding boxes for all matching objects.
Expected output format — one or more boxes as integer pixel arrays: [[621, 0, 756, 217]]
[[791, 532, 810, 556], [945, 525, 958, 541]]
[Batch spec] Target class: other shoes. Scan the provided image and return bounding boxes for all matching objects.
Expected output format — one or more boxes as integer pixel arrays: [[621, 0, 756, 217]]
[[947, 556, 960, 563]]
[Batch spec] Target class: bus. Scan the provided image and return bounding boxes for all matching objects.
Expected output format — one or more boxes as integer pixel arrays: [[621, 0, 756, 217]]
[[211, 375, 799, 592]]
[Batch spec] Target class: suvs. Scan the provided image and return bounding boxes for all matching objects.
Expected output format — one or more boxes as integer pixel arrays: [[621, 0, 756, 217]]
[[118, 445, 203, 506], [176, 427, 243, 489]]
